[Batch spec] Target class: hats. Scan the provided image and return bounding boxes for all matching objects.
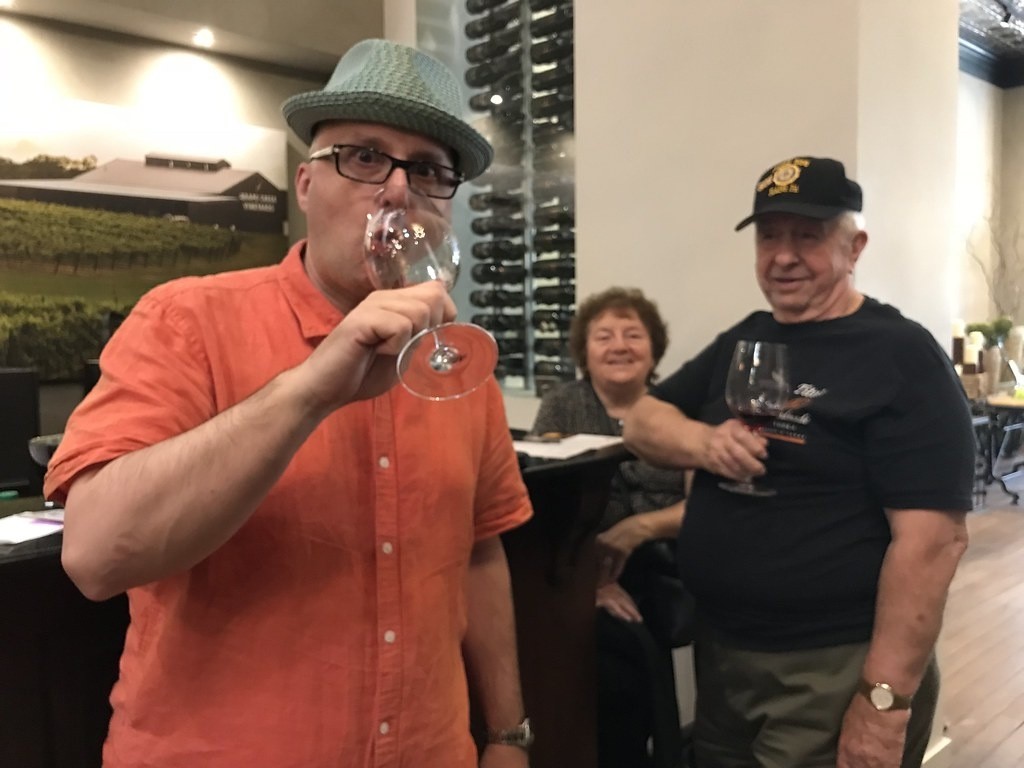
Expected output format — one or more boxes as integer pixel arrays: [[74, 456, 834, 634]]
[[734, 154, 863, 232], [282, 38, 494, 181]]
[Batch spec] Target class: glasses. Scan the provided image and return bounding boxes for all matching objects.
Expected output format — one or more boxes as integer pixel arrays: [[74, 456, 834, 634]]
[[308, 144, 465, 201]]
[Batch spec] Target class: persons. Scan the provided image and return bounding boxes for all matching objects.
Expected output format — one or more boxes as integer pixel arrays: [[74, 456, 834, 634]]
[[46, 40, 536, 768], [536, 283, 697, 765], [623, 157, 978, 768]]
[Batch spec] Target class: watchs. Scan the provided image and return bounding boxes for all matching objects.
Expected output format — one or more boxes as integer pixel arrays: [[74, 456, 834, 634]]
[[487, 719, 533, 750], [855, 674, 911, 713]]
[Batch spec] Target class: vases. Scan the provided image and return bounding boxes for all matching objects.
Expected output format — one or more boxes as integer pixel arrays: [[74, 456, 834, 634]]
[[981, 344, 1002, 394]]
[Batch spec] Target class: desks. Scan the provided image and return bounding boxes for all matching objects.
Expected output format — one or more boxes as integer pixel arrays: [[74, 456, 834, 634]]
[[0, 425, 635, 767], [974, 387, 1024, 506]]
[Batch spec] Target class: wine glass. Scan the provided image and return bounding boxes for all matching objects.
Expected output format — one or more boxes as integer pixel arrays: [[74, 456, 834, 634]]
[[360, 184, 499, 400], [717, 339, 788, 496]]
